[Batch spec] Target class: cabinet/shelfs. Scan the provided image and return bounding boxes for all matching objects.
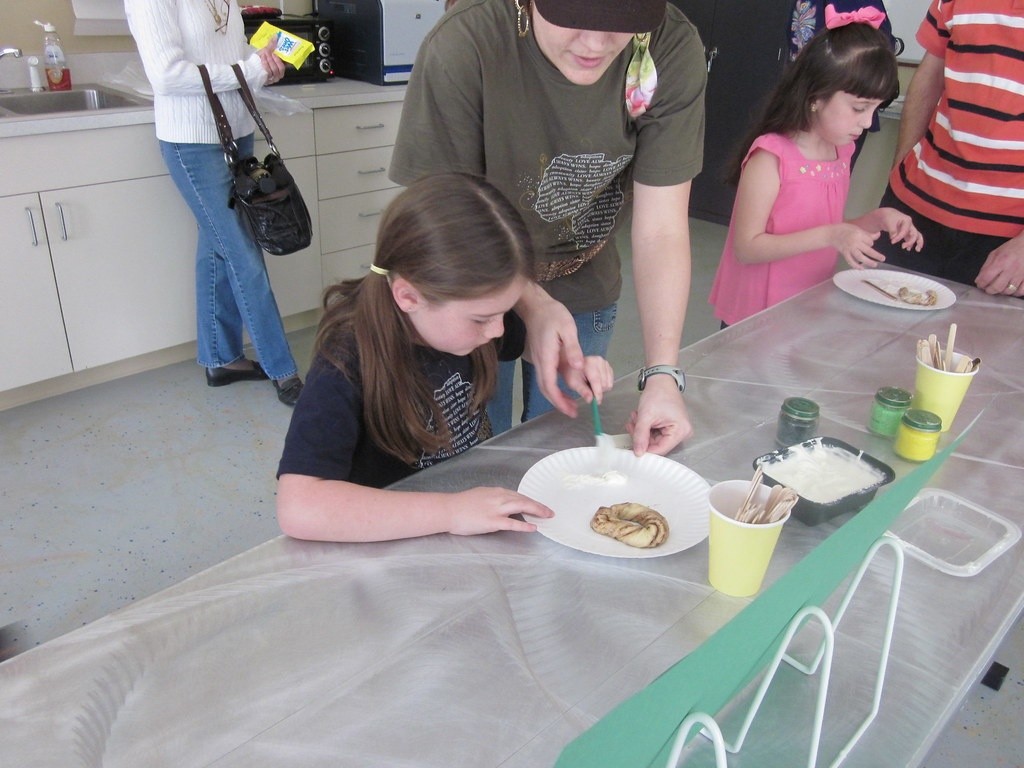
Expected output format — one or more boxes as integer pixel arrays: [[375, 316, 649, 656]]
[[252, 110, 317, 325], [0, 111, 197, 399], [311, 97, 406, 306]]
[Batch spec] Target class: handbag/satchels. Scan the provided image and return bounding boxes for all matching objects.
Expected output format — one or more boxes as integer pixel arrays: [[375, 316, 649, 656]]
[[195, 63, 313, 256]]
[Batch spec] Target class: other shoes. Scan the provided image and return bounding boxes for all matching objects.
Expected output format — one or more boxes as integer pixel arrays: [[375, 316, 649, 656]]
[[206, 360, 269, 387], [272, 377, 305, 406]]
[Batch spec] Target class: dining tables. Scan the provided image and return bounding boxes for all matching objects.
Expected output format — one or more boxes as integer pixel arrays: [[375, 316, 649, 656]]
[[0, 258, 1024, 768]]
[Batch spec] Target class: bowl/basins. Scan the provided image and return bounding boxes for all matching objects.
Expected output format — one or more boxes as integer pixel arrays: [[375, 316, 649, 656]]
[[753, 435, 895, 525]]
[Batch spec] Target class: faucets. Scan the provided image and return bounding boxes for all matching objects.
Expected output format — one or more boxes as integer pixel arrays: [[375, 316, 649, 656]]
[[0, 48, 23, 59]]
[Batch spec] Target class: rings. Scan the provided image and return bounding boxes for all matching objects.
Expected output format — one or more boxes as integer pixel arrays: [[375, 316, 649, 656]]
[[1007, 284, 1017, 291]]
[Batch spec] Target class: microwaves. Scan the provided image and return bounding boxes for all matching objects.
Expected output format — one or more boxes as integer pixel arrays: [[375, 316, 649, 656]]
[[243, 13, 333, 87]]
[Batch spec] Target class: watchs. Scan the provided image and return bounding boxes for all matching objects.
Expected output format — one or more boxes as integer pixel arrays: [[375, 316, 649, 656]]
[[637, 365, 686, 393]]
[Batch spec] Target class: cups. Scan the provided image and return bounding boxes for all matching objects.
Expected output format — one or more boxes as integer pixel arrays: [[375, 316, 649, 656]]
[[914, 349, 979, 432], [707, 480, 791, 597]]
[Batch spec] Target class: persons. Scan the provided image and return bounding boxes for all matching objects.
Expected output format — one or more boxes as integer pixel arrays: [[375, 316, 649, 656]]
[[389, 0, 709, 457], [708, 4, 924, 330], [123, 0, 304, 406], [873, 0, 1024, 300], [276, 172, 614, 542]]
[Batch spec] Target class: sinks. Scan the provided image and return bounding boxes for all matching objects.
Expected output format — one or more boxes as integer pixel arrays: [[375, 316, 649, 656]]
[[0, 84, 145, 114]]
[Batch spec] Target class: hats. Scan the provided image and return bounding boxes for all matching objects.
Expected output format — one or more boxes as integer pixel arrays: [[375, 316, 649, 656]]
[[534, 0, 667, 33]]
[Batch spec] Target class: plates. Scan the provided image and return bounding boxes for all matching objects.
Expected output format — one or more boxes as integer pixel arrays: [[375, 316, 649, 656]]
[[517, 444, 710, 558], [832, 268, 956, 311]]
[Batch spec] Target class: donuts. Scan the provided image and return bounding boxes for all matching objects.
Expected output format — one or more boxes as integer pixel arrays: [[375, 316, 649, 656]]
[[591, 502, 670, 548], [899, 287, 937, 306]]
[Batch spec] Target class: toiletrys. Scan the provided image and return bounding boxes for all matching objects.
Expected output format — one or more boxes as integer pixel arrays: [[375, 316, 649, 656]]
[[32, 17, 72, 92]]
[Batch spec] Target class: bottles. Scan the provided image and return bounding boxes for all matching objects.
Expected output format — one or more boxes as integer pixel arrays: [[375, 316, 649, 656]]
[[33, 20, 71, 91], [867, 385, 913, 438], [774, 396, 821, 447], [893, 409, 942, 463]]
